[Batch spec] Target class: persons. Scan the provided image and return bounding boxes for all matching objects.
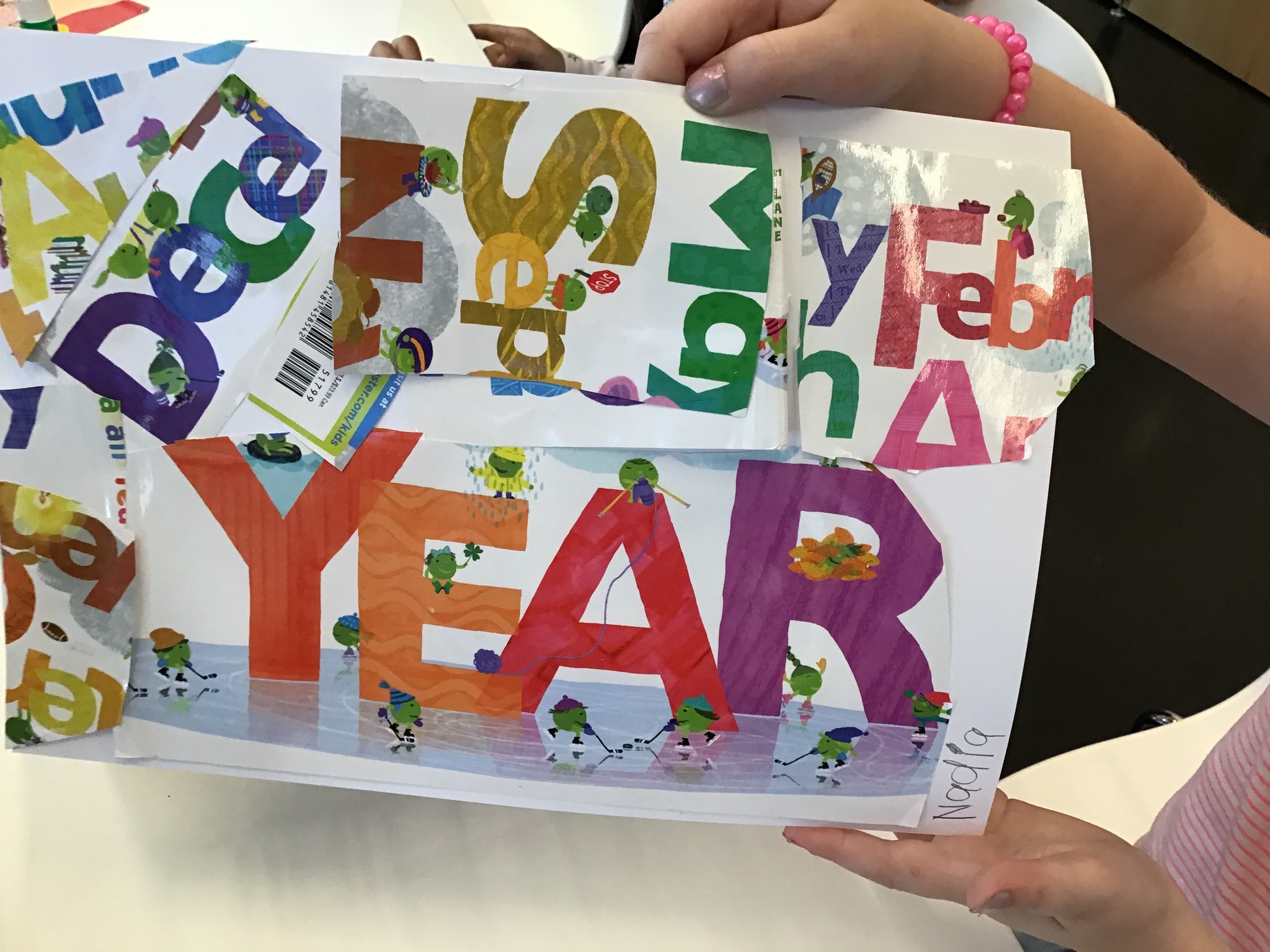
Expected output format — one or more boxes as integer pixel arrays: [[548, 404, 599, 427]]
[[370, 24, 635, 78], [633, 0, 1270, 952]]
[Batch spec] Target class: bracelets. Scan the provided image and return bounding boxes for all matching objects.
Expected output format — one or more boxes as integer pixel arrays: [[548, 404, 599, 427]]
[[964, 14, 1033, 123]]
